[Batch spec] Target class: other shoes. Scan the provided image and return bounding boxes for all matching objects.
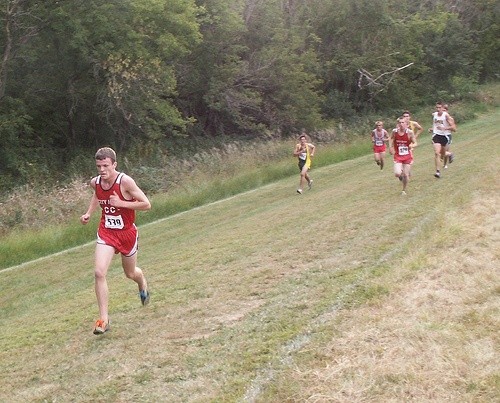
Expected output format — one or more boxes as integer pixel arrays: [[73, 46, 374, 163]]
[[375, 157, 453, 195], [297, 189, 302, 194], [308, 180, 312, 188]]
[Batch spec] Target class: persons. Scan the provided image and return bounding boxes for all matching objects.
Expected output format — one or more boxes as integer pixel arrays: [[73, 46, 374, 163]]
[[80, 147, 151, 336], [439, 102, 457, 164], [292, 136, 316, 195], [428, 101, 457, 178], [370, 121, 392, 171], [401, 111, 423, 139], [389, 116, 418, 196]]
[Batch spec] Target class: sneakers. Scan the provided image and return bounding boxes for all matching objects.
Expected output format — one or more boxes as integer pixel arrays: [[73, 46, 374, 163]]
[[93, 319, 110, 334], [139, 287, 149, 306]]
